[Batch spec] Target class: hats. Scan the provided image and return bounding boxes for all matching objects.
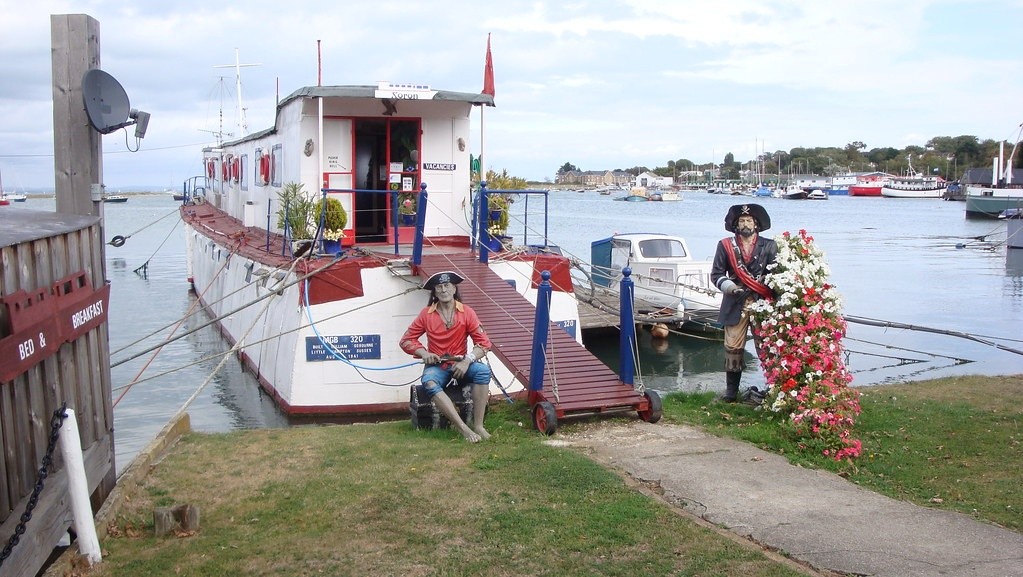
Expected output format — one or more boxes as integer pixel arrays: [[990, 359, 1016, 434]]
[[725, 203, 771, 232], [422, 271, 465, 290]]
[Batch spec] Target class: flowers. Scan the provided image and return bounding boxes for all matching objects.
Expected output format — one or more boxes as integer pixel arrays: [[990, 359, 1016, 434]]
[[399, 199, 415, 215], [314, 196, 348, 242], [488, 224, 505, 241]]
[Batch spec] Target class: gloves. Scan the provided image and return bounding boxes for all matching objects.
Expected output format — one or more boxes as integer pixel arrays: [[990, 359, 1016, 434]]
[[721, 280, 738, 296]]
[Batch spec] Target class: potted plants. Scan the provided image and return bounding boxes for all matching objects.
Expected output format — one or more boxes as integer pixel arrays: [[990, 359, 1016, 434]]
[[275, 181, 316, 257], [488, 197, 503, 220]]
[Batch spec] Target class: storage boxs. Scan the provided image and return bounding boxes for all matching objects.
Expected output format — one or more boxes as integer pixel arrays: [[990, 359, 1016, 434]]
[[409, 384, 488, 430]]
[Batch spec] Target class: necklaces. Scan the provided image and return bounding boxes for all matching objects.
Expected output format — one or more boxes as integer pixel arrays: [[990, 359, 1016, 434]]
[[440, 305, 454, 327]]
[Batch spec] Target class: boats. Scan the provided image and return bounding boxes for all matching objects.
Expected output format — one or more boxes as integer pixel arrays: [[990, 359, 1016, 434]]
[[708, 184, 828, 200], [179, 33, 584, 418], [173, 194, 189, 200], [104, 196, 128, 202], [3, 190, 26, 203], [585, 231, 753, 344], [613, 186, 683, 202], [849, 186, 949, 197]]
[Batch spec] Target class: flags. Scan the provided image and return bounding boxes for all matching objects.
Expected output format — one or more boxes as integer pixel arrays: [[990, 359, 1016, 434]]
[[482, 36, 495, 98]]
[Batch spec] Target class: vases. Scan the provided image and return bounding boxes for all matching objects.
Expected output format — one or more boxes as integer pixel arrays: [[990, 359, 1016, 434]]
[[488, 236, 504, 252], [323, 239, 342, 255], [402, 212, 417, 226]]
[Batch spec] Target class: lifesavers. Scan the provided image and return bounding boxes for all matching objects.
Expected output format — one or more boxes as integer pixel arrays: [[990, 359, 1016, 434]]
[[233, 158, 239, 182], [208, 162, 214, 177], [223, 162, 226, 181], [260, 148, 269, 185]]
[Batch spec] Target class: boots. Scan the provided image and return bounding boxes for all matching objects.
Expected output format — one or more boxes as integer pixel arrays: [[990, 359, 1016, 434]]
[[722, 348, 746, 402]]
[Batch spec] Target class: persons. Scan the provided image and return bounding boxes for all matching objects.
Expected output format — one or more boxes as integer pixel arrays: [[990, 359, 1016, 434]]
[[711, 204, 780, 403], [399, 271, 493, 443]]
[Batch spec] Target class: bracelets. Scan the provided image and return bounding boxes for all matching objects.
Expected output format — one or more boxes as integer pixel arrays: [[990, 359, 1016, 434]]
[[468, 354, 477, 363]]
